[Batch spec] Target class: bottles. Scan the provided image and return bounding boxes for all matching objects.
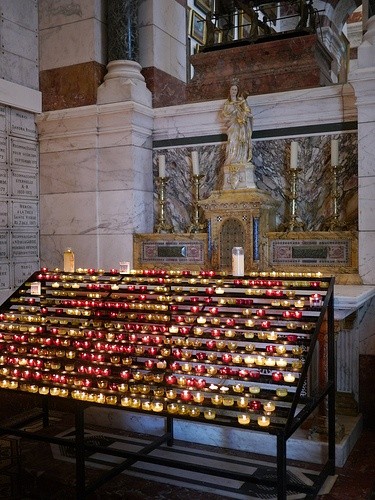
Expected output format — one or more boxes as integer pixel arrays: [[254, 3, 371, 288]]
[[232, 247, 245, 276], [64, 248, 74, 272]]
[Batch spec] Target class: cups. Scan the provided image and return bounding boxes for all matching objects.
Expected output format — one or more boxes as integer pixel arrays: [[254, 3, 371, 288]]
[[31, 282, 41, 295], [119, 261, 130, 274]]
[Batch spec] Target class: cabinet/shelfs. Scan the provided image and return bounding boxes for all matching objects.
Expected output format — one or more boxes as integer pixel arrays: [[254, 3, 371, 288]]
[[0, 251, 336, 500]]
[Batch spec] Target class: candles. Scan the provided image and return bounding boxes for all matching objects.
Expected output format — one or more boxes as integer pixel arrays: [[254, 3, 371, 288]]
[[290, 141, 298, 168], [0, 269, 325, 426], [158, 155, 165, 176], [331, 140, 338, 166], [192, 151, 199, 174]]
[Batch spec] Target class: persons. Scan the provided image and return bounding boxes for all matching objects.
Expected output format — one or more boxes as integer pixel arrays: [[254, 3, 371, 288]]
[[221, 77, 253, 162]]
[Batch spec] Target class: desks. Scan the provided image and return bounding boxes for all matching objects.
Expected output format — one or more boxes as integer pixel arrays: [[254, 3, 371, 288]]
[[321, 285, 375, 415]]
